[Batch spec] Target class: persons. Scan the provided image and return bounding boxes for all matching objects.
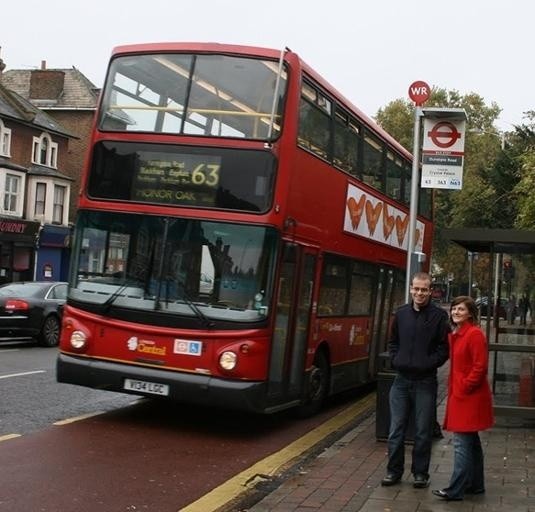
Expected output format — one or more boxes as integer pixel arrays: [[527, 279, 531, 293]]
[[432, 295, 495, 499], [381, 272, 451, 488], [504, 294, 535, 327]]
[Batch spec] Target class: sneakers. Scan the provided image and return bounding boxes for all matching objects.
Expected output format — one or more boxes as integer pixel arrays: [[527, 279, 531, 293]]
[[465, 485, 486, 495]]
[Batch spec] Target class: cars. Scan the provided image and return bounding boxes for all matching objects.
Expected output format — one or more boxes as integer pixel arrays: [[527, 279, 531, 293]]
[[474, 296, 520, 321], [1, 281, 70, 347]]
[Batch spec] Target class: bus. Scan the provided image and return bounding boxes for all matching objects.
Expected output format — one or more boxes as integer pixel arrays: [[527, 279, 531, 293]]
[[56, 42, 434, 419]]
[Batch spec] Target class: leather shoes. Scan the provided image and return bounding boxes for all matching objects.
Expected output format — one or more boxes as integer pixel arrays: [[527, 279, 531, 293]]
[[412, 472, 431, 487], [431, 487, 465, 501], [381, 473, 402, 486]]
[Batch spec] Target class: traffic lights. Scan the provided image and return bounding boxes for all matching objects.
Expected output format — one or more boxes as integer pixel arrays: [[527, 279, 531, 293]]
[[503, 259, 512, 282]]
